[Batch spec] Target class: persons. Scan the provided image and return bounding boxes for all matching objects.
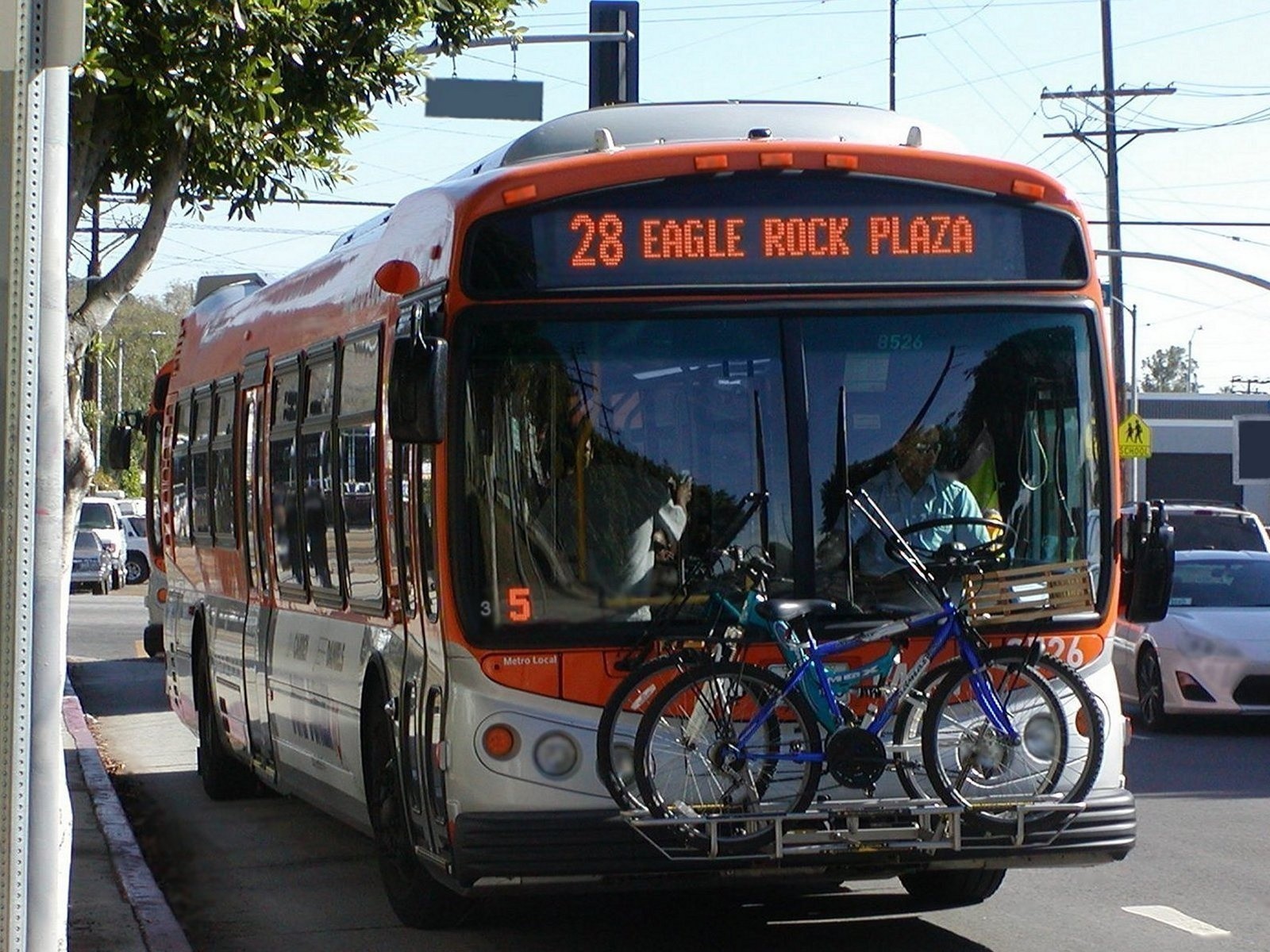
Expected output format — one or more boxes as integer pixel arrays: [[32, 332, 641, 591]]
[[819, 422, 992, 612], [533, 418, 694, 621]]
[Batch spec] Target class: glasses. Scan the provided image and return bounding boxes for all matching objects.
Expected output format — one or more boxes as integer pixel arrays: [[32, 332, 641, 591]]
[[905, 441, 941, 455]]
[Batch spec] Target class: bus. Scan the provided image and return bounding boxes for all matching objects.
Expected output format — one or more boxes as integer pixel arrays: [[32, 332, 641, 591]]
[[144, 95, 1183, 930]]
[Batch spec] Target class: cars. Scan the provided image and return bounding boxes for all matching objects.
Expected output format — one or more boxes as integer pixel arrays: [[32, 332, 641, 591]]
[[1109, 550, 1270, 732], [98, 490, 154, 584], [71, 531, 115, 594]]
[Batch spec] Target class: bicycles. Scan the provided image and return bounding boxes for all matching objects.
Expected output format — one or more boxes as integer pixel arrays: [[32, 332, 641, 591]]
[[592, 536, 1106, 843]]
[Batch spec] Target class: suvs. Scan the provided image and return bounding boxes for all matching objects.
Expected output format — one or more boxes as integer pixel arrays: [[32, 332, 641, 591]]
[[1083, 501, 1270, 589], [80, 497, 132, 587]]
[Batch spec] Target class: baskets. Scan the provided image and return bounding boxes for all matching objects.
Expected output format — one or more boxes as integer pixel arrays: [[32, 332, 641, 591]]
[[962, 559, 1096, 626]]
